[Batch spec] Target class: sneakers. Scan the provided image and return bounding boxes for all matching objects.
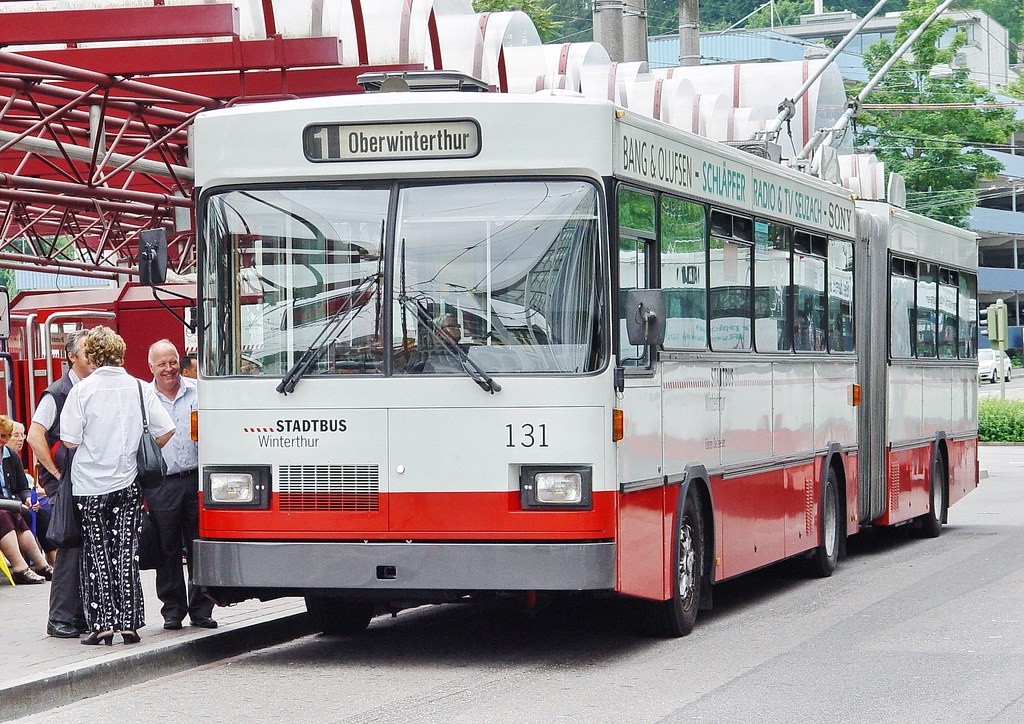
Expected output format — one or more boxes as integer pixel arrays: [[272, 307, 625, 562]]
[[164, 620, 182, 629], [190, 618, 217, 629]]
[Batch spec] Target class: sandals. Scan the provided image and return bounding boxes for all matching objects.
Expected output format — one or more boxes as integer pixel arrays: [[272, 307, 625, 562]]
[[36, 565, 53, 581], [13, 567, 46, 584]]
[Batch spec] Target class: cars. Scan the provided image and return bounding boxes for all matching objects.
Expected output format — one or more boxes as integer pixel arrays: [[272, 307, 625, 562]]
[[977, 347, 1012, 383]]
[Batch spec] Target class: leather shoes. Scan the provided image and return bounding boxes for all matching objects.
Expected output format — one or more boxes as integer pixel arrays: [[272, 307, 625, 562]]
[[46, 620, 80, 638], [74, 621, 89, 633]]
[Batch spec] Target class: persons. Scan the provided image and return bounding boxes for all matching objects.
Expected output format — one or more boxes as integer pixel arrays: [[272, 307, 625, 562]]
[[0, 325, 218, 646]]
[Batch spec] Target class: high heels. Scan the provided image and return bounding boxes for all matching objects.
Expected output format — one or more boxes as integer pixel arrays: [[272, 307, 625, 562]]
[[121, 631, 140, 644], [81, 632, 115, 646]]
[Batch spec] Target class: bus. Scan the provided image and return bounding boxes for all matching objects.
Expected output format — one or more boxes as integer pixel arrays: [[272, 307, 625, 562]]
[[136, 67, 981, 636], [619, 250, 976, 362]]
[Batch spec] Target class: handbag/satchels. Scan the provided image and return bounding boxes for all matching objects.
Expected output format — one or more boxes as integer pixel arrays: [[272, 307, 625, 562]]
[[45, 449, 84, 548], [136, 379, 167, 490]]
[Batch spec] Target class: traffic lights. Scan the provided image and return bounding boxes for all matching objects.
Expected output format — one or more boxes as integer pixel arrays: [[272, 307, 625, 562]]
[[980, 308, 997, 342]]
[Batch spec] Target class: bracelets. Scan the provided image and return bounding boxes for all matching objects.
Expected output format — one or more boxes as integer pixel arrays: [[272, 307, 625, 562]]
[[53, 470, 58, 475]]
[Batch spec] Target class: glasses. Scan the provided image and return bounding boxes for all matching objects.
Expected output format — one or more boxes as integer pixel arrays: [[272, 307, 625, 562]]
[[10, 435, 26, 439]]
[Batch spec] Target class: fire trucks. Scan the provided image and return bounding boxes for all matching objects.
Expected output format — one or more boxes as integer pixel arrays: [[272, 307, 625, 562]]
[[0, 283, 264, 503]]
